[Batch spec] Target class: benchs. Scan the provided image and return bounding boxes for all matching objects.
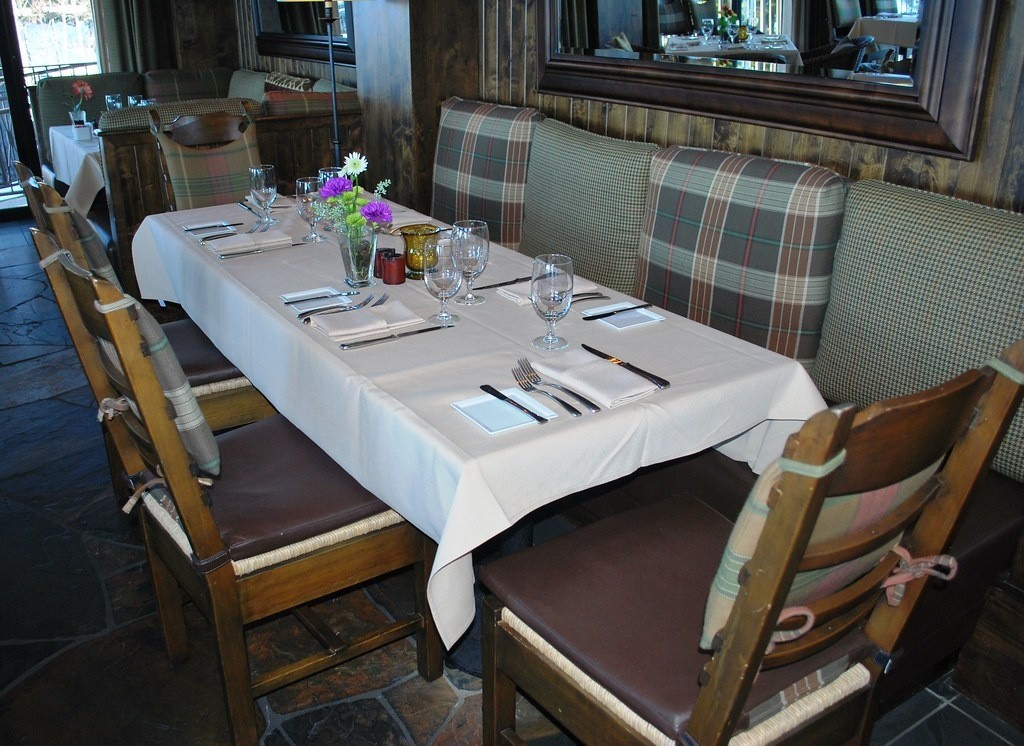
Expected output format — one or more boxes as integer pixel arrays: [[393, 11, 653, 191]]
[[23, 65, 363, 266]]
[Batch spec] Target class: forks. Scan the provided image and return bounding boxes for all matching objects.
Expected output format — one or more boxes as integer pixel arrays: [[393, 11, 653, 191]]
[[511, 367, 583, 418], [296, 293, 375, 320], [518, 357, 601, 413], [302, 292, 390, 324]]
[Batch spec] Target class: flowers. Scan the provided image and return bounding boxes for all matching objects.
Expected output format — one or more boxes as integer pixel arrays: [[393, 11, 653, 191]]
[[63, 80, 93, 119], [716, 4, 740, 33], [319, 151, 392, 273]]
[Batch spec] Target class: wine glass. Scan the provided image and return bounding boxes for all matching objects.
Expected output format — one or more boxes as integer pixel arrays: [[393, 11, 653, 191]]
[[530, 253, 574, 352], [449, 220, 490, 306], [399, 223, 441, 281], [700, 19, 714, 46], [726, 20, 740, 44], [248, 164, 280, 226], [421, 237, 464, 325], [295, 166, 344, 244]]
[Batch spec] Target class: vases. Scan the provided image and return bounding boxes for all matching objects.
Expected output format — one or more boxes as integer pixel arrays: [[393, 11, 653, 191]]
[[335, 227, 376, 288], [69, 110, 87, 125]]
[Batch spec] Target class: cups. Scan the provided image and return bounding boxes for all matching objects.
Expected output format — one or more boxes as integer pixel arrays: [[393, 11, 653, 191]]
[[737, 26, 748, 40], [104, 94, 123, 111], [127, 94, 158, 107], [374, 248, 406, 286]]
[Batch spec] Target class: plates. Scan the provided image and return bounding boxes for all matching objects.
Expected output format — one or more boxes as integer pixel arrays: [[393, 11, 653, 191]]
[[762, 35, 788, 44], [278, 286, 354, 312], [724, 45, 744, 48], [245, 192, 293, 208], [668, 44, 688, 51], [183, 219, 237, 239], [580, 301, 666, 330], [449, 387, 560, 435]]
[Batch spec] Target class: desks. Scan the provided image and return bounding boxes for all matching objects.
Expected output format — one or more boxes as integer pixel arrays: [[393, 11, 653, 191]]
[[132, 187, 829, 654], [661, 33, 804, 74], [848, 14, 919, 62], [49, 124, 106, 220]]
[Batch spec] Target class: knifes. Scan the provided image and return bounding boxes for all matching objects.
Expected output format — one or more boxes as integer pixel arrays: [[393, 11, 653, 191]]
[[217, 242, 306, 259], [339, 325, 454, 350], [471, 271, 558, 291], [581, 343, 671, 389], [237, 201, 263, 219], [582, 303, 654, 320], [480, 384, 548, 425], [185, 223, 243, 231], [283, 291, 360, 306]]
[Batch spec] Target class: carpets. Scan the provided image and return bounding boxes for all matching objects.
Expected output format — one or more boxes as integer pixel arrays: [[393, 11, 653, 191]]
[[363, 510, 580, 678]]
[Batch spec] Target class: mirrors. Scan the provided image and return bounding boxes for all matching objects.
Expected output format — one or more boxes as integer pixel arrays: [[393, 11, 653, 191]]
[[535, 0, 1005, 162]]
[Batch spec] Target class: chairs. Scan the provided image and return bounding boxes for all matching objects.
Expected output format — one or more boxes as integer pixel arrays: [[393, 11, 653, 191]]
[[827, 0, 907, 63], [145, 100, 262, 213], [28, 225, 445, 746], [11, 159, 280, 502], [800, 36, 874, 79], [659, 0, 718, 35], [429, 96, 1024, 746]]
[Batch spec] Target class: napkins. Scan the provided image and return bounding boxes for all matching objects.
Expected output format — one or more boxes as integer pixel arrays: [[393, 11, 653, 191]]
[[531, 346, 659, 411], [204, 229, 292, 256], [311, 299, 427, 342], [496, 273, 598, 307], [391, 208, 433, 230], [243, 194, 300, 215]]
